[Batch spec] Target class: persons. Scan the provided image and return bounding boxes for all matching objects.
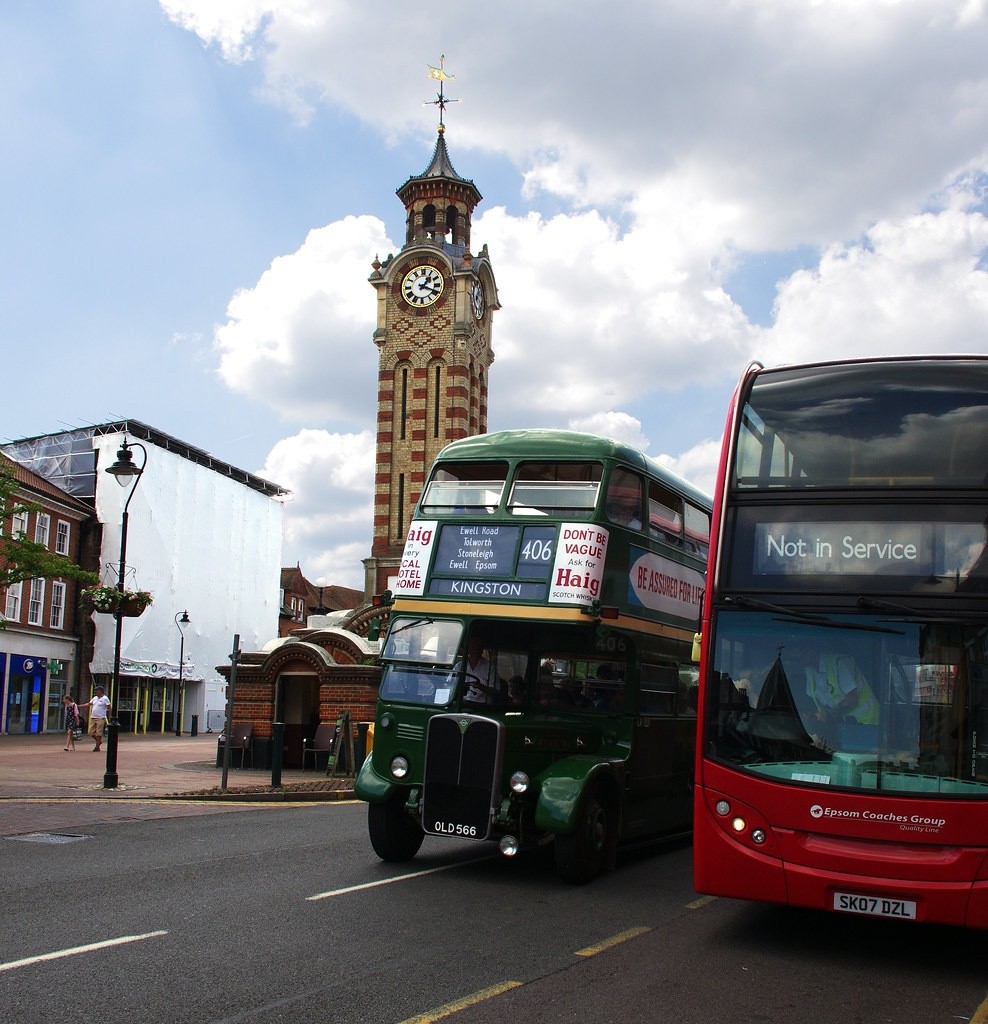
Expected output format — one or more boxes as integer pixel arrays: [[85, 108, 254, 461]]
[[442, 643, 627, 715], [62, 695, 79, 751], [792, 639, 882, 753], [452, 489, 489, 515], [77, 686, 112, 751]]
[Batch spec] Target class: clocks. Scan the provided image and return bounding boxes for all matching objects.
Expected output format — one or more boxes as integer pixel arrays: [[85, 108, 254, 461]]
[[471, 275, 485, 319], [400, 265, 444, 308]]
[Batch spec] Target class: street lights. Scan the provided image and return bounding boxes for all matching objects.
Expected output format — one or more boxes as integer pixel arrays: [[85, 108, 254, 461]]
[[175, 610, 190, 734], [104, 437, 148, 787]]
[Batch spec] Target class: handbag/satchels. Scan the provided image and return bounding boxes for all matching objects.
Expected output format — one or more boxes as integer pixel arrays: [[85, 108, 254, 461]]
[[72, 723, 83, 741]]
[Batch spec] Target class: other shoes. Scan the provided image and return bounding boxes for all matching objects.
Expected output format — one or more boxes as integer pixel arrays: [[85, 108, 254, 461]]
[[92, 749, 100, 751], [70, 747, 75, 751], [97, 742, 102, 747], [64, 748, 69, 751]]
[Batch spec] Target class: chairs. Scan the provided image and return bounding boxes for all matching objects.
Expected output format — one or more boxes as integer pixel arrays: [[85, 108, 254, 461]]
[[302, 725, 336, 771], [216, 723, 254, 771]]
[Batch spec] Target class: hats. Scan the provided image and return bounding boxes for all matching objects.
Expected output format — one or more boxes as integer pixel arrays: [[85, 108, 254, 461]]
[[94, 686, 104, 691]]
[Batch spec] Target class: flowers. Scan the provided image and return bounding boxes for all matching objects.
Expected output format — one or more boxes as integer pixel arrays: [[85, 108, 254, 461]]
[[113, 591, 155, 616], [77, 585, 115, 611]]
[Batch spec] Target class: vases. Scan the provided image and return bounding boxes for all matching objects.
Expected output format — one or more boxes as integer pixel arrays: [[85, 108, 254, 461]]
[[92, 598, 114, 613], [124, 603, 146, 617]]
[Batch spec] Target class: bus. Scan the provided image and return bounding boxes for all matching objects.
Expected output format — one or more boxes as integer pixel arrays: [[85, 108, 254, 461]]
[[356, 428, 741, 883], [691, 350, 988, 928]]
